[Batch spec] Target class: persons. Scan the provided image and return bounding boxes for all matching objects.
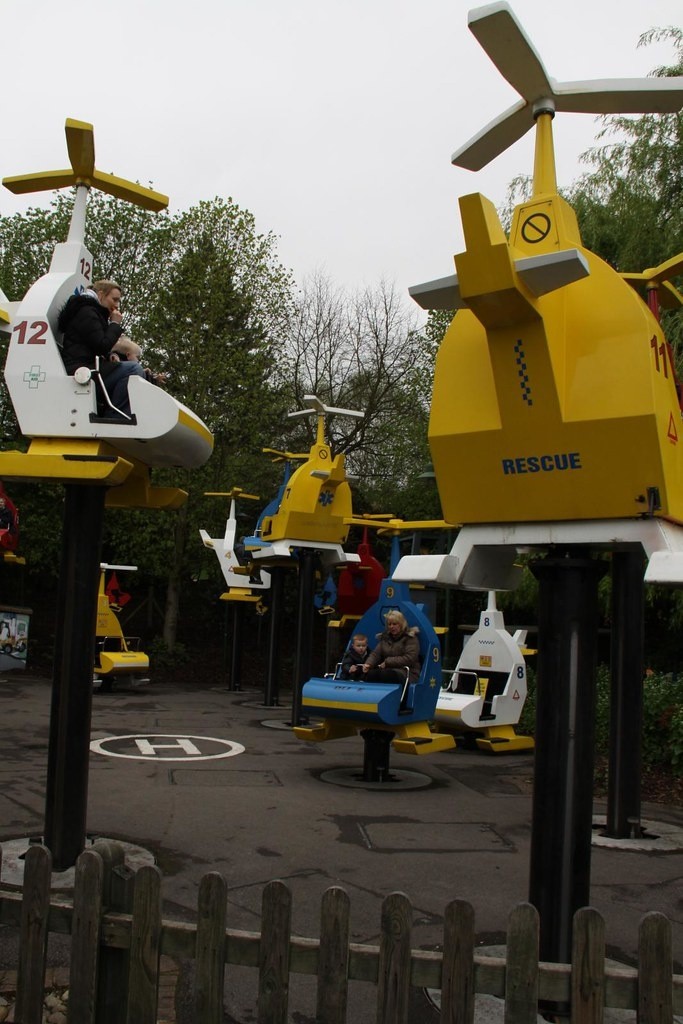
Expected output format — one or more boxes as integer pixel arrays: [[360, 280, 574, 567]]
[[341, 610, 418, 684], [57, 280, 167, 416]]
